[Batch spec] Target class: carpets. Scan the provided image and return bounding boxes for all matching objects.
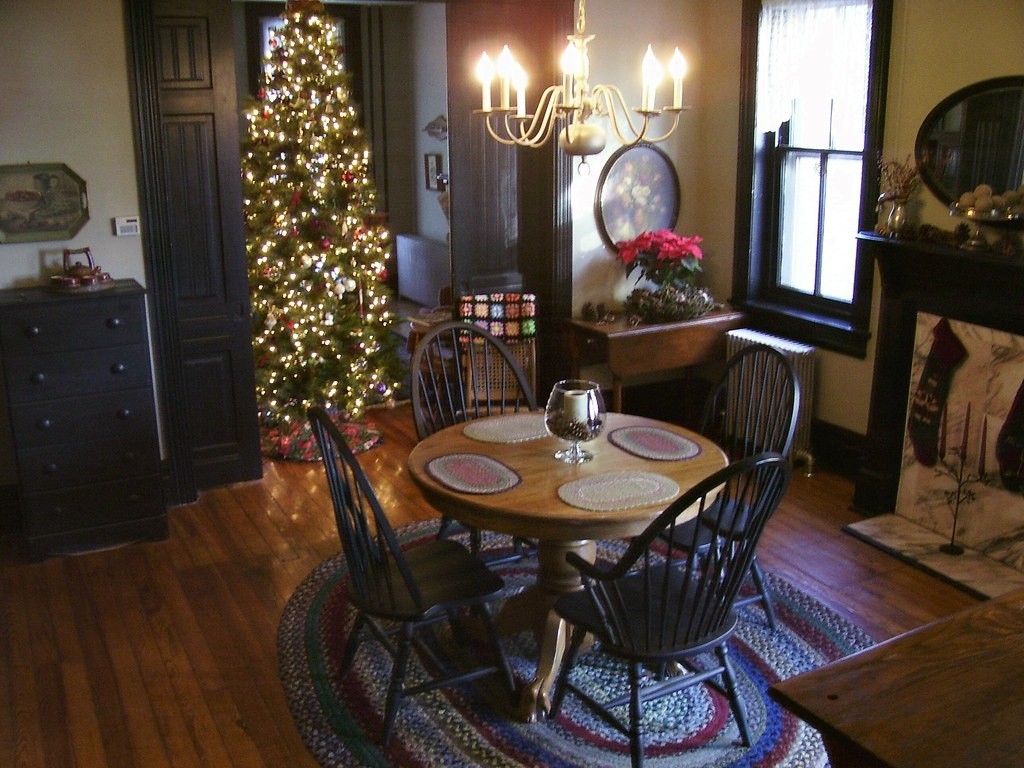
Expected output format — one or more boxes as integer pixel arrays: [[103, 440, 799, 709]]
[[275, 518, 879, 768]]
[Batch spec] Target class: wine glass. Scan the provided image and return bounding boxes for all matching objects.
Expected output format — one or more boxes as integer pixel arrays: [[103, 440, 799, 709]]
[[544, 379, 607, 464]]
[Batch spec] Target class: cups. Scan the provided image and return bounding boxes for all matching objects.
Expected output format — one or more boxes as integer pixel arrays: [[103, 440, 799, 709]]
[[50, 273, 111, 287]]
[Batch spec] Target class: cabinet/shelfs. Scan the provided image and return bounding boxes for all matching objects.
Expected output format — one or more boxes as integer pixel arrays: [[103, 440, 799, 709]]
[[1, 278, 173, 565]]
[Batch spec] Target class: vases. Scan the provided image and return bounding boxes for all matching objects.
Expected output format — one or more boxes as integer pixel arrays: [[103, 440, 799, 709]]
[[888, 198, 911, 234]]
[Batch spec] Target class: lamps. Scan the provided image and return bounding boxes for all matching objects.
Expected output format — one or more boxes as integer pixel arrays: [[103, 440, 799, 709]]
[[472, 0, 687, 178]]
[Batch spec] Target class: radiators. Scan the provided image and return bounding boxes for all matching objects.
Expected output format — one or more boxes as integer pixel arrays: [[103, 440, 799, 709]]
[[722, 328, 817, 480], [397, 233, 450, 308]]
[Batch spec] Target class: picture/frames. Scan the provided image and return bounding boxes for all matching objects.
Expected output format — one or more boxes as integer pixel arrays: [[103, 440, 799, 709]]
[[593, 141, 683, 252], [424, 152, 444, 191], [0, 162, 91, 244]]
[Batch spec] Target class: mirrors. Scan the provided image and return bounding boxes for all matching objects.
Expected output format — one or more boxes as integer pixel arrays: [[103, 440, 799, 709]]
[[914, 75, 1024, 230]]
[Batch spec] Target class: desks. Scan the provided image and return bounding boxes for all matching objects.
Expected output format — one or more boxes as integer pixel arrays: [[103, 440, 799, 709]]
[[407, 311, 455, 422], [407, 410, 729, 659], [766, 584, 1024, 768], [565, 307, 745, 413]]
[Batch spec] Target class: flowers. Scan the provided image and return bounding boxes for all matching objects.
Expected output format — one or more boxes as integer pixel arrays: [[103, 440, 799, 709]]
[[875, 151, 921, 203], [614, 230, 702, 291]]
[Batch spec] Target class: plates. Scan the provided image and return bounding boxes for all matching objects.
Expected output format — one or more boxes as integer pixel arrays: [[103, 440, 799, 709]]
[[948, 202, 1021, 253]]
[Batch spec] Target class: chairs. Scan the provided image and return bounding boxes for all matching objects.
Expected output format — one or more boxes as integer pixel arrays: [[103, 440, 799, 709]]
[[659, 343, 801, 632], [547, 451, 791, 768], [457, 294, 538, 422], [409, 321, 540, 568], [305, 404, 519, 747]]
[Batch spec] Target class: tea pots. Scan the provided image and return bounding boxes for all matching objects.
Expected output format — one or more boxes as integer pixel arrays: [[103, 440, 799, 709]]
[[63, 247, 101, 277]]
